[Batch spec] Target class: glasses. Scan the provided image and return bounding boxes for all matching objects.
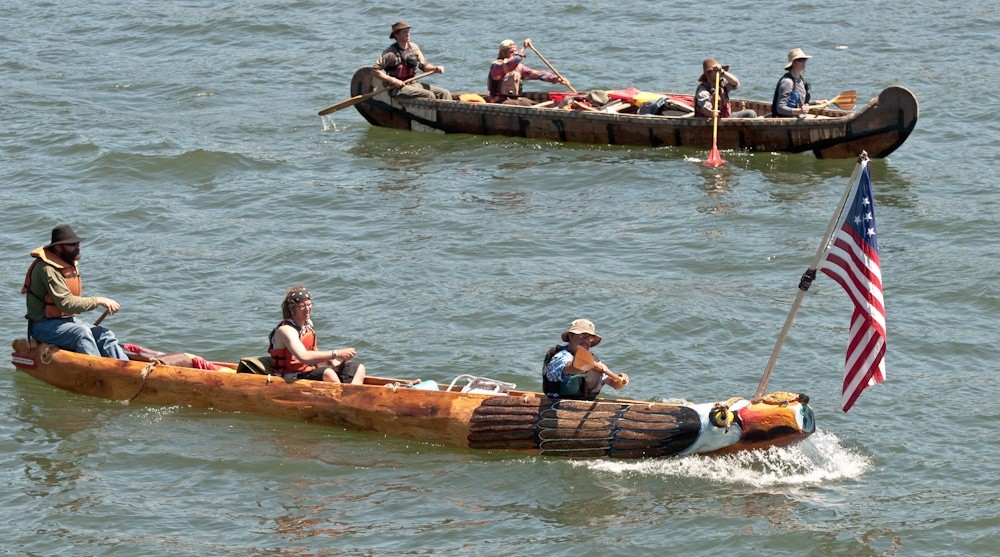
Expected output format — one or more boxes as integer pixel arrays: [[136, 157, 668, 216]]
[[575, 334, 594, 342], [293, 302, 314, 311], [61, 243, 79, 247]]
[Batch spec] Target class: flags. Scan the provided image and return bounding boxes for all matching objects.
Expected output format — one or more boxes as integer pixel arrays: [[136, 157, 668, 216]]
[[818, 160, 888, 413]]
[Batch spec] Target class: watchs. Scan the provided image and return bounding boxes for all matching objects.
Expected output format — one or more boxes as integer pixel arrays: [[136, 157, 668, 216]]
[[332, 350, 337, 359]]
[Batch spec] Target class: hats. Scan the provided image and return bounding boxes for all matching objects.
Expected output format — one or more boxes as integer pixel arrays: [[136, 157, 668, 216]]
[[698, 58, 730, 82], [390, 20, 412, 39], [784, 48, 812, 70], [561, 319, 603, 347], [43, 224, 86, 248], [500, 39, 517, 52]]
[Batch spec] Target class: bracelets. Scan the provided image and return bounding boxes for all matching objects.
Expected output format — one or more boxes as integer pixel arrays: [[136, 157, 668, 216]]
[[721, 70, 724, 75]]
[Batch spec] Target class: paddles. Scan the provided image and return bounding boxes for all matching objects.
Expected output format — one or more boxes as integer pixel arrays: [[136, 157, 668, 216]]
[[809, 89, 858, 111], [707, 71, 722, 163], [317, 69, 438, 117], [573, 343, 626, 389], [528, 41, 576, 92], [94, 307, 111, 326]]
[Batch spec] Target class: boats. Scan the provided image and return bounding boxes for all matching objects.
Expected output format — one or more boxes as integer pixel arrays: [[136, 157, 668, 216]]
[[8, 333, 817, 463], [348, 66, 920, 159]]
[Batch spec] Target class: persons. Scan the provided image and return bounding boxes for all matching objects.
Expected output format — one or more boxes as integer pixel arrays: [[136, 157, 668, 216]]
[[268, 286, 365, 385], [771, 48, 832, 118], [542, 319, 628, 400], [21, 224, 129, 360], [372, 20, 452, 100], [487, 40, 570, 106], [694, 57, 758, 119]]
